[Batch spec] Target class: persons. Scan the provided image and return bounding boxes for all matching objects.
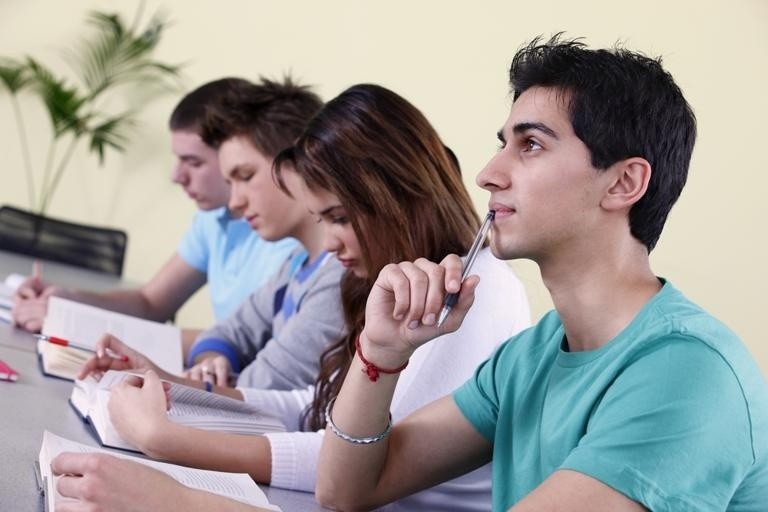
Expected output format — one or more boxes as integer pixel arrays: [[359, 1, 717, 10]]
[[11, 77, 298, 362], [48, 30, 768, 512], [75, 81, 535, 511], [178, 67, 354, 390]]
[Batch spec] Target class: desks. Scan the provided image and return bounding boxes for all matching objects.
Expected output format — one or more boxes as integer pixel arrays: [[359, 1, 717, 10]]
[[1, 247, 384, 510]]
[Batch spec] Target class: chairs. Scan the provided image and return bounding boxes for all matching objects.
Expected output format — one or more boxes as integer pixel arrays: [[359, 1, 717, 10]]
[[0, 206, 127, 283]]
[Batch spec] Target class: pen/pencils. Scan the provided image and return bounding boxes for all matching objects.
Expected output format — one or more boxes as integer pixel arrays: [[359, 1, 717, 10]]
[[33, 333, 129, 363], [436, 209, 496, 328], [33, 258, 42, 290], [206, 371, 240, 378]]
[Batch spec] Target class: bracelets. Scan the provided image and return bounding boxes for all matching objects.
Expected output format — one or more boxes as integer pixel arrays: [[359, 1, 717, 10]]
[[323, 395, 393, 446], [354, 334, 410, 382]]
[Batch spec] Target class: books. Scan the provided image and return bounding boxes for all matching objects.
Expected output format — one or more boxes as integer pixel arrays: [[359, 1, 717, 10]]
[[0, 272, 26, 323], [67, 367, 287, 455], [35, 293, 183, 384], [31, 429, 281, 511], [0, 360, 21, 383]]
